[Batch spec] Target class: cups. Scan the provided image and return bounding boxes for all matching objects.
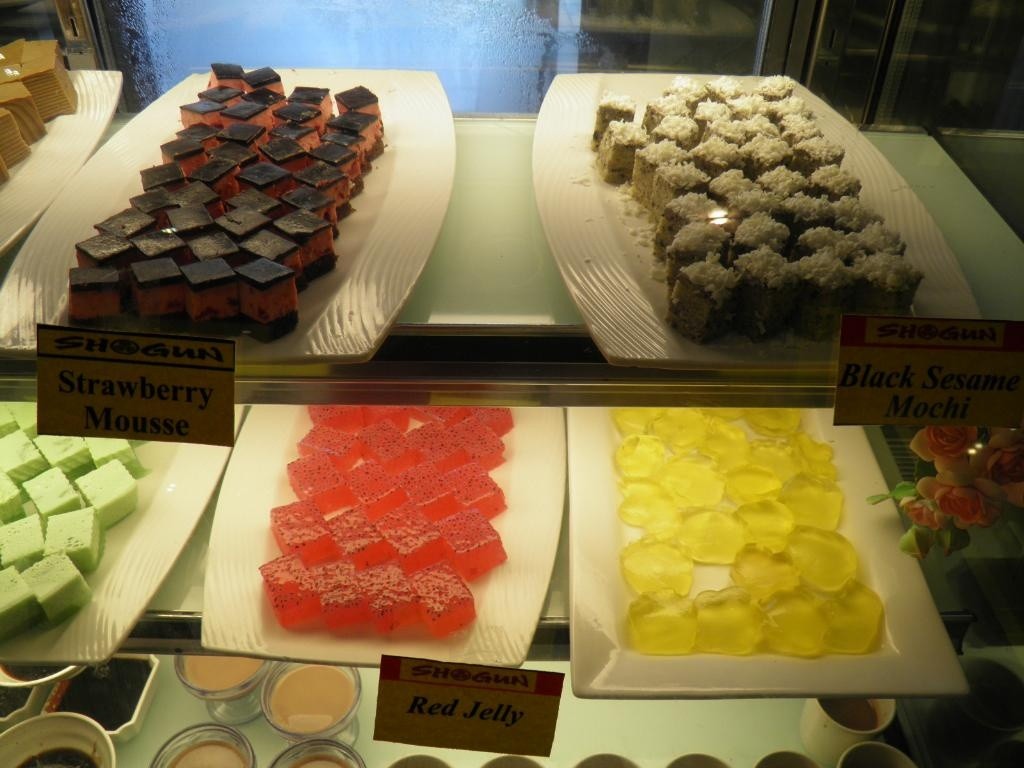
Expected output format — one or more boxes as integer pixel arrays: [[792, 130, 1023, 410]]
[[378, 702, 915, 768], [148, 722, 257, 768], [267, 737, 366, 768], [945, 654, 1024, 768]]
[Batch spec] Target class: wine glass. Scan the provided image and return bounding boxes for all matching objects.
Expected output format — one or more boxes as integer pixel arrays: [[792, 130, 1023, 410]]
[[260, 659, 363, 749], [172, 651, 272, 725]]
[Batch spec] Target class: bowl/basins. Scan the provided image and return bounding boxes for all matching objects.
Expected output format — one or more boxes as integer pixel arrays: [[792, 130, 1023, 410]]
[[0, 654, 160, 768]]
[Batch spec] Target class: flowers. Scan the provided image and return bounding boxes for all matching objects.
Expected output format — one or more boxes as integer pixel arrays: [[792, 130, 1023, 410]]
[[866, 426, 1024, 559]]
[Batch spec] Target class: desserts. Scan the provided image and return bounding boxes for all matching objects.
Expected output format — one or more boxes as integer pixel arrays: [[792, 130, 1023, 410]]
[[260, 402, 515, 637], [0, 400, 147, 645], [591, 74, 927, 346], [68, 64, 386, 343], [606, 407, 885, 657], [0, 38, 79, 185]]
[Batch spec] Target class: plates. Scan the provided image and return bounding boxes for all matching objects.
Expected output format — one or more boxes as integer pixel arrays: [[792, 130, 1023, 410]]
[[0, 403, 249, 669], [199, 401, 569, 669], [1, 66, 457, 363], [530, 72, 983, 371], [566, 405, 972, 700], [0, 69, 125, 259]]
